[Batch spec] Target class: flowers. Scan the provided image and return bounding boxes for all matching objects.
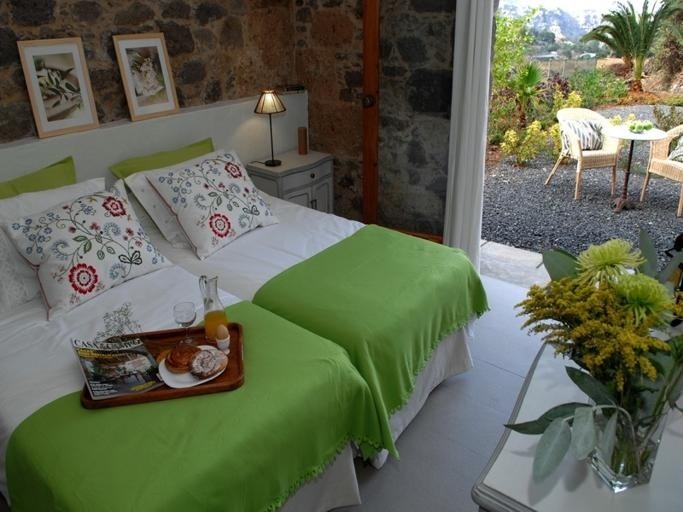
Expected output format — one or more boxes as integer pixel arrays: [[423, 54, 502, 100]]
[[500, 224, 683, 487]]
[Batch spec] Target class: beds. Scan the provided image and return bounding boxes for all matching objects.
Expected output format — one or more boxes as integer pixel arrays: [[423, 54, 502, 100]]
[[1, 156, 372, 511], [109, 128, 491, 472]]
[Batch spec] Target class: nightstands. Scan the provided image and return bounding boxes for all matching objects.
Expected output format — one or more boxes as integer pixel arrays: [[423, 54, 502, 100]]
[[245, 149, 338, 215]]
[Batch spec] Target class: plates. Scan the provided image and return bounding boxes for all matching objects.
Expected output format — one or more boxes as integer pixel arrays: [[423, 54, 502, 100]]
[[157, 346, 228, 389]]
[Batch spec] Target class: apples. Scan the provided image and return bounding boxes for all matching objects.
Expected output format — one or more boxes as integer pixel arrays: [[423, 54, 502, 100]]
[[630, 120, 652, 132]]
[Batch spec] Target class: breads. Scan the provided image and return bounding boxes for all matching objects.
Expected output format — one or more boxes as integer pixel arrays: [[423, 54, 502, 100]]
[[165, 345, 200, 374], [190, 350, 229, 379]]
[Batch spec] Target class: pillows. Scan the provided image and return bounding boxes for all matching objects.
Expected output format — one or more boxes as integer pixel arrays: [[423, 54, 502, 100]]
[[667, 135, 683, 165], [110, 140, 281, 261], [2, 156, 175, 322], [562, 115, 605, 153]]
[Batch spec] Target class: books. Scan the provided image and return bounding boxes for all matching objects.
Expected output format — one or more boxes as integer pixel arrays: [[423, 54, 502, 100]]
[[70, 337, 164, 402]]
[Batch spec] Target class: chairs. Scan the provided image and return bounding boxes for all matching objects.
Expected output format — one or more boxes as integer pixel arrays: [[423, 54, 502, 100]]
[[542, 106, 622, 201], [638, 122, 683, 221]]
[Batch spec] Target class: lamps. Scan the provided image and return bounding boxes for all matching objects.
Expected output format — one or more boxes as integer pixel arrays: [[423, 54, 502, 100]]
[[254, 87, 288, 169]]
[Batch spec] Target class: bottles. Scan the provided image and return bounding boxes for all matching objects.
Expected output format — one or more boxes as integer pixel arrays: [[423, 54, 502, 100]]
[[297, 127, 307, 155]]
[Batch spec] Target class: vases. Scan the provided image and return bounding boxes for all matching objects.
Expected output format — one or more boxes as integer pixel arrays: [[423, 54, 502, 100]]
[[588, 383, 669, 497]]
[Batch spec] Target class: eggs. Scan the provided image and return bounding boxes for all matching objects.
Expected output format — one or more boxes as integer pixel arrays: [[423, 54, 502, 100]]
[[216, 325, 228, 339]]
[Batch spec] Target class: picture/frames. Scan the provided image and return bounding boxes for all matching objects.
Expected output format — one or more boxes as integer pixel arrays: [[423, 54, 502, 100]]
[[16, 36, 100, 139], [111, 31, 182, 123]]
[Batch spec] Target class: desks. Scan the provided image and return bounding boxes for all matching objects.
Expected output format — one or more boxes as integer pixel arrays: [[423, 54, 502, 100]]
[[467, 342, 682, 512], [599, 124, 668, 217]]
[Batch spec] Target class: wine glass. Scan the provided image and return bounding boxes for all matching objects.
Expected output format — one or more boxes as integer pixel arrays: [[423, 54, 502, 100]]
[[217, 336, 231, 355], [195, 274, 227, 341], [171, 300, 199, 346]]
